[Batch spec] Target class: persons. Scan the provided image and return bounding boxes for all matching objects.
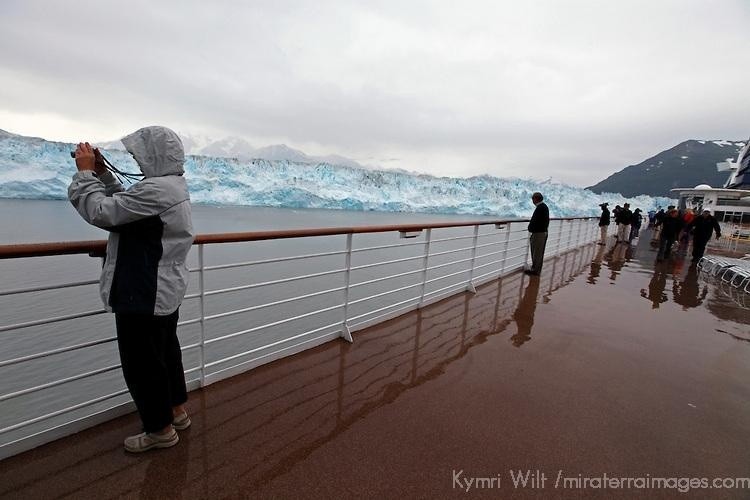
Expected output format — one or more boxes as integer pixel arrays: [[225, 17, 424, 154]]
[[612, 203, 721, 262], [524, 192, 549, 275], [640, 262, 668, 310], [586, 243, 637, 285], [138, 426, 191, 500], [511, 276, 539, 346], [67, 126, 194, 453], [598, 203, 610, 245], [673, 260, 708, 311]]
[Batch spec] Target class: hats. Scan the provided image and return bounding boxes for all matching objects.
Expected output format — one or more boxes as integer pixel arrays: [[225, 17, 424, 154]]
[[635, 208, 643, 212], [599, 203, 609, 207], [702, 207, 711, 213]]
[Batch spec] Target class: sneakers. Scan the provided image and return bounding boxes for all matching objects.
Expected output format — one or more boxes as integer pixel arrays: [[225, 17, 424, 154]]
[[657, 253, 687, 258], [690, 257, 704, 263], [524, 269, 542, 277], [597, 242, 607, 247], [122, 424, 180, 455], [173, 411, 194, 432], [617, 240, 631, 245]]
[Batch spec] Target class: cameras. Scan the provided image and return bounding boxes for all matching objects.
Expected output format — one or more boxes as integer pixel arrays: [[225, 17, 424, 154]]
[[71, 148, 97, 163]]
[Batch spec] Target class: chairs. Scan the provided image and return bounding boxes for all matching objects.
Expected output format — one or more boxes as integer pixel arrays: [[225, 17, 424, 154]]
[[697, 273, 749, 310], [694, 251, 749, 297]]
